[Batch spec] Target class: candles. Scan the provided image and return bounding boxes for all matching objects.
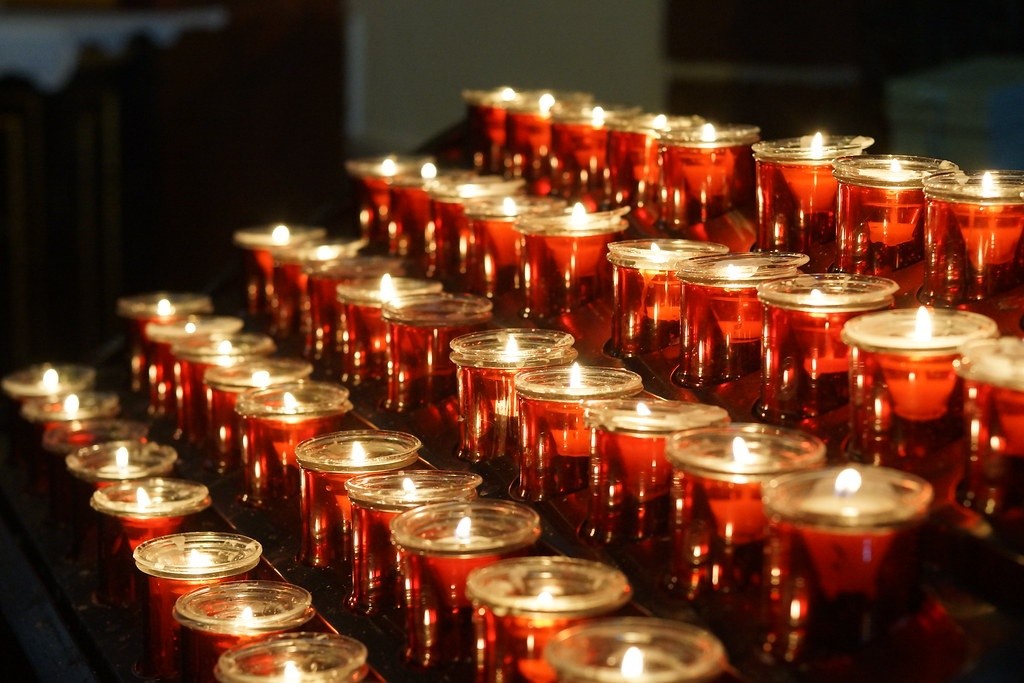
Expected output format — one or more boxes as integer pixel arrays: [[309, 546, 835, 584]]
[[0, 90, 1024, 683]]
